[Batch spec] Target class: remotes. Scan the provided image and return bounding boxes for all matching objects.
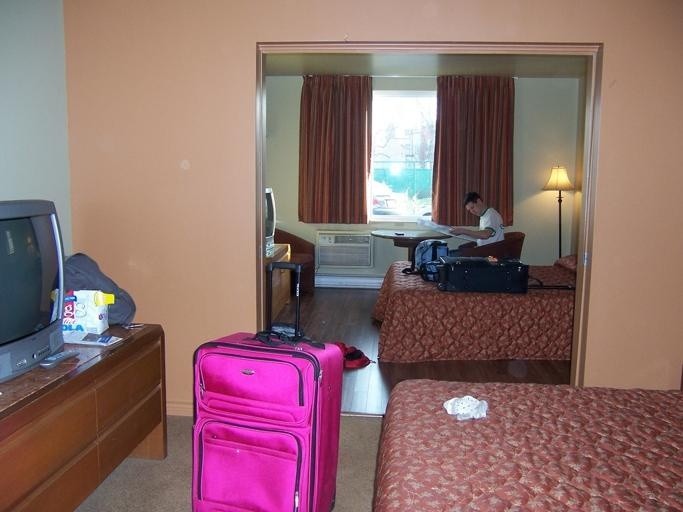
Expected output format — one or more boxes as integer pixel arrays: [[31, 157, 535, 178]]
[[39, 349, 80, 368]]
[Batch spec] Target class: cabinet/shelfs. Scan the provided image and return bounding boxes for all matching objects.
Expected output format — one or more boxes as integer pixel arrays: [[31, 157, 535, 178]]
[[265, 244, 291, 331], [0, 322, 167, 512]]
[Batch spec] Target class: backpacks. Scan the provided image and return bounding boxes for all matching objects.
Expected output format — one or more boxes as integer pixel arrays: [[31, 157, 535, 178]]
[[411, 239, 449, 282]]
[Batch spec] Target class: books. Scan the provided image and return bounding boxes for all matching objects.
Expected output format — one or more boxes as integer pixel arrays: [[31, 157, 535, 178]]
[[61, 329, 123, 346]]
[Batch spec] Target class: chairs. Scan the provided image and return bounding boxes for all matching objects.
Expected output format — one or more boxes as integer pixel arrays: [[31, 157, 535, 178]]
[[458, 232, 525, 257], [274, 228, 315, 294]]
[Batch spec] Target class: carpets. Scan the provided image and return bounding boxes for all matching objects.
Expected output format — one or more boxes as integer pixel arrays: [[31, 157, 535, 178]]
[[74, 415, 383, 512]]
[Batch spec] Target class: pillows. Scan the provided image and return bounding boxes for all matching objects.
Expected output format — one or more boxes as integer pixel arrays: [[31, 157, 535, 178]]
[[554, 254, 577, 274]]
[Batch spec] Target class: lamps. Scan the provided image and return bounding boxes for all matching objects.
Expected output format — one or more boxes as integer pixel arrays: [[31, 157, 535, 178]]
[[542, 165, 575, 260]]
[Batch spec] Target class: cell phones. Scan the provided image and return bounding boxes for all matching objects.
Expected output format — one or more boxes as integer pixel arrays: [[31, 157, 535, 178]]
[[395, 232, 405, 235]]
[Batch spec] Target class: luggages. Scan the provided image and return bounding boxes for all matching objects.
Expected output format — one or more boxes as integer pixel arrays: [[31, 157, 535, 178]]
[[433, 255, 575, 294], [191, 262, 344, 512]]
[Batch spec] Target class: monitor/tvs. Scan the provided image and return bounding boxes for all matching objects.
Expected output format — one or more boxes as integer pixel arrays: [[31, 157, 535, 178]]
[[264, 187, 277, 250], [0, 198, 66, 384]]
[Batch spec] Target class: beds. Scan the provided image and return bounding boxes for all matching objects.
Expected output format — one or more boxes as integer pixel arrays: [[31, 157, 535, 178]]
[[372, 260, 576, 364], [373, 378, 683, 512]]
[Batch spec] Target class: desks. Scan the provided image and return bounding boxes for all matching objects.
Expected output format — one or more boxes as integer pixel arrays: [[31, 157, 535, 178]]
[[371, 230, 452, 261]]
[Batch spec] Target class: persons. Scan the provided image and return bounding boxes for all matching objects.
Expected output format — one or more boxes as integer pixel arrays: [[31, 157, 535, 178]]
[[447, 191, 505, 247]]
